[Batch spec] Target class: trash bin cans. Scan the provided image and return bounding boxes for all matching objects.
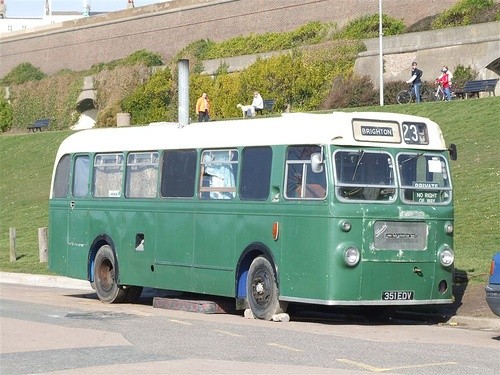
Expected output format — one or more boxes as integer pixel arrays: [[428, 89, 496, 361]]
[[117, 113, 131, 127]]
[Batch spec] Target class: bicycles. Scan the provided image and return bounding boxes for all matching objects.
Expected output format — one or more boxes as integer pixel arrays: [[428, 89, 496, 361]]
[[434, 64, 454, 101], [396, 81, 441, 106]]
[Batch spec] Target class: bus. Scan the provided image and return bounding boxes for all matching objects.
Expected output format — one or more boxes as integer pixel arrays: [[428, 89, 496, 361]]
[[45, 111, 458, 322]]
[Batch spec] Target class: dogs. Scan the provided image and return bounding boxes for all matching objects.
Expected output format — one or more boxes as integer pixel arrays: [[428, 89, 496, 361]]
[[236, 104, 256, 118]]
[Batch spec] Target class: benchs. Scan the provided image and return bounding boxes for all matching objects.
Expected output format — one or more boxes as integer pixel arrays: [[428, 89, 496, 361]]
[[255, 100, 275, 115], [454, 79, 499, 98], [28, 120, 50, 132]]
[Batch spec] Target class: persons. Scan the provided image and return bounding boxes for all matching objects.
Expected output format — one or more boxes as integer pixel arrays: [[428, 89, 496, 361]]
[[434, 67, 452, 101], [406, 61, 422, 103], [436, 65, 454, 89], [252, 90, 263, 110], [435, 53, 440, 56], [196, 92, 210, 122]]
